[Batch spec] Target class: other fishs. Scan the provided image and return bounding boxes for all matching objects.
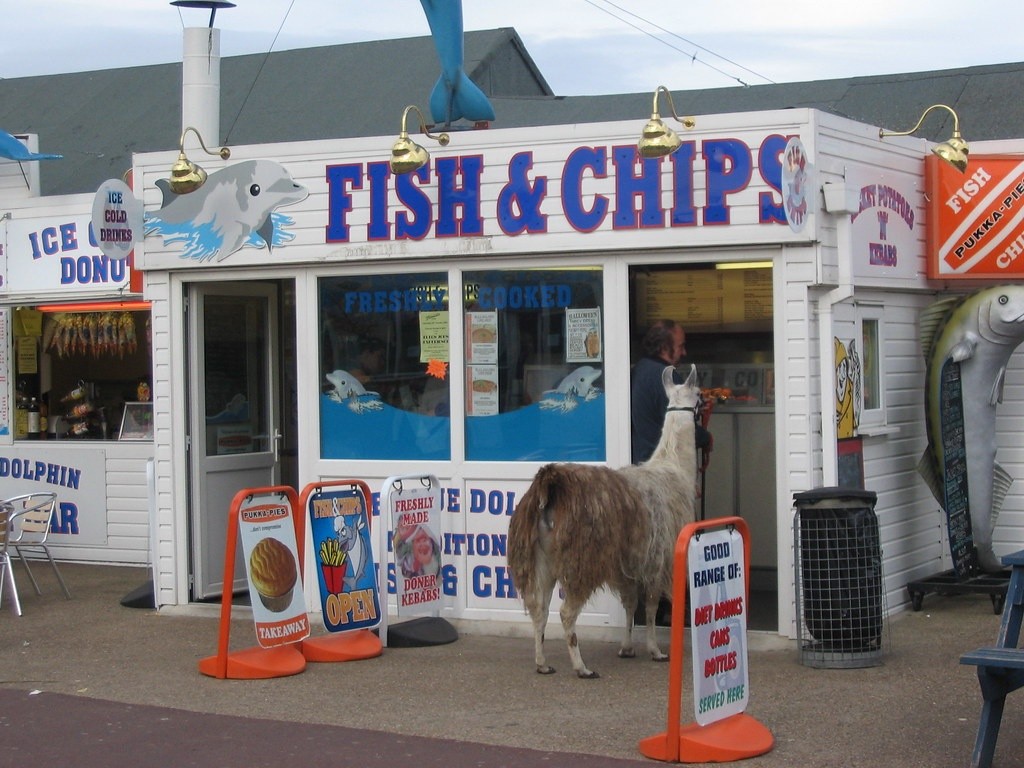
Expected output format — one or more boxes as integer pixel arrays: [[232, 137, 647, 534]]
[[905, 283, 1023, 583]]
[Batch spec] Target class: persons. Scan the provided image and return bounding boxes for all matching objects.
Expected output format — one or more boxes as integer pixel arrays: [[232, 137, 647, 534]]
[[631, 318, 712, 629], [344, 334, 387, 400]]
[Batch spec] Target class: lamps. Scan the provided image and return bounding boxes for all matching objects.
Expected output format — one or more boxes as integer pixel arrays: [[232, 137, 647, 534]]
[[637, 85, 695, 159], [390, 105, 450, 174], [879, 104, 969, 175], [169, 127, 230, 193]]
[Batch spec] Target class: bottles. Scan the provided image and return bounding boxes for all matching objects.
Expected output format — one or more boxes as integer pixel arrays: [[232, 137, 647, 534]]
[[64, 420, 91, 437], [40, 400, 48, 439], [16, 397, 28, 440], [100, 417, 110, 439], [28, 397, 41, 440], [61, 402, 92, 421], [60, 387, 87, 402]]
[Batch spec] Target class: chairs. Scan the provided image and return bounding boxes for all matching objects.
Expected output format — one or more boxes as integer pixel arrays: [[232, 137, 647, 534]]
[[0, 491, 73, 617]]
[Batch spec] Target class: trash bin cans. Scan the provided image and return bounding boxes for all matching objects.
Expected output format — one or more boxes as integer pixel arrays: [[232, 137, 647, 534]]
[[790, 480, 890, 668]]
